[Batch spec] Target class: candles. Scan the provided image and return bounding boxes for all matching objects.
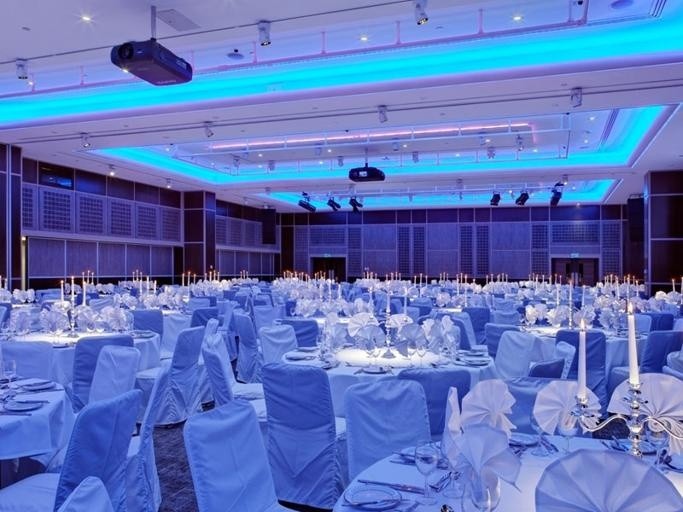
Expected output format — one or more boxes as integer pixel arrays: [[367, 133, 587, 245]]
[[60, 270, 94, 306], [21, 237, 26, 290]]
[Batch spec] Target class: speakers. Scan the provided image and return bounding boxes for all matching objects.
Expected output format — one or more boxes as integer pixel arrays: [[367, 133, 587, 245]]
[[261, 209, 276, 245], [626, 198, 645, 242]]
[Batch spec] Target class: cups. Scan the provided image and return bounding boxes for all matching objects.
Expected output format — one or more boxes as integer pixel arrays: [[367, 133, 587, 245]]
[[113, 294, 181, 313]]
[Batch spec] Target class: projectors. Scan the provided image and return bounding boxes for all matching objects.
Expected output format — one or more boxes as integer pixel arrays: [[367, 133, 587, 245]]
[[349, 167, 385, 182], [111, 40, 192, 85]]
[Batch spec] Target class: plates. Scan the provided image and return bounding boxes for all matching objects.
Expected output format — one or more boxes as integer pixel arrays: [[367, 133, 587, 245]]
[[612, 437, 652, 453], [462, 350, 485, 356], [399, 445, 427, 460], [130, 329, 156, 339], [341, 486, 403, 510], [506, 432, 538, 445], [467, 359, 490, 367], [22, 381, 56, 392], [363, 366, 390, 374], [5, 398, 42, 410]]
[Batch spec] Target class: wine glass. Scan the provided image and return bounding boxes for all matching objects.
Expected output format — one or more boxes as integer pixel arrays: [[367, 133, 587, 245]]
[[0, 309, 134, 343], [3, 361, 17, 393], [644, 421, 671, 475], [284, 300, 360, 321], [416, 446, 438, 506], [557, 411, 579, 455], [314, 328, 460, 373], [432, 300, 436, 308], [444, 299, 448, 309], [518, 313, 626, 338]]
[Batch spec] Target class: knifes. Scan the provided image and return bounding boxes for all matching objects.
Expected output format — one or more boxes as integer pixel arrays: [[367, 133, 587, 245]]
[[338, 498, 418, 508], [538, 435, 560, 454], [356, 478, 425, 495]]
